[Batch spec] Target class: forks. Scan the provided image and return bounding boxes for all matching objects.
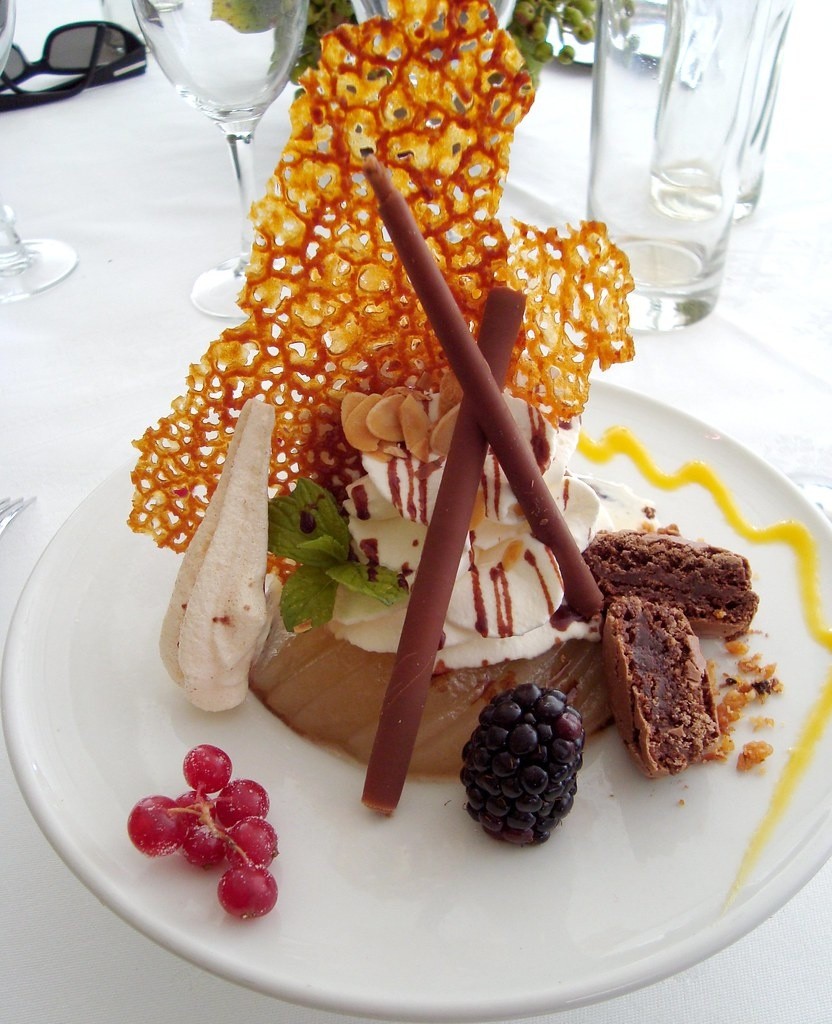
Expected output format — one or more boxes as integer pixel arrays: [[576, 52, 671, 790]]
[[0, 497, 36, 536]]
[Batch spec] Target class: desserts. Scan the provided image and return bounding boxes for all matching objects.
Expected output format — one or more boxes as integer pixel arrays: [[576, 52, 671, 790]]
[[581, 531, 757, 636], [605, 598, 726, 773], [157, 395, 618, 781]]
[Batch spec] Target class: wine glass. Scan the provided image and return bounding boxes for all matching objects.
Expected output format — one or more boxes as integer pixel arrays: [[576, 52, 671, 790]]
[[131, 0, 310, 317], [0, 1, 82, 300]]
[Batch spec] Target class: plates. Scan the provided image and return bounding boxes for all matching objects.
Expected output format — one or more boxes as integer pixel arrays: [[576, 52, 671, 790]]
[[0, 356, 832, 1024]]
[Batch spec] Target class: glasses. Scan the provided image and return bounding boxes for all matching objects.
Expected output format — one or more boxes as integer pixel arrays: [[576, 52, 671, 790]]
[[0, 21, 148, 112]]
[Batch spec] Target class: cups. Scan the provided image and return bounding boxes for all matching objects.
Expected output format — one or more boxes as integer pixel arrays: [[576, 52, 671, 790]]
[[650, 0, 795, 222], [587, 0, 774, 332]]
